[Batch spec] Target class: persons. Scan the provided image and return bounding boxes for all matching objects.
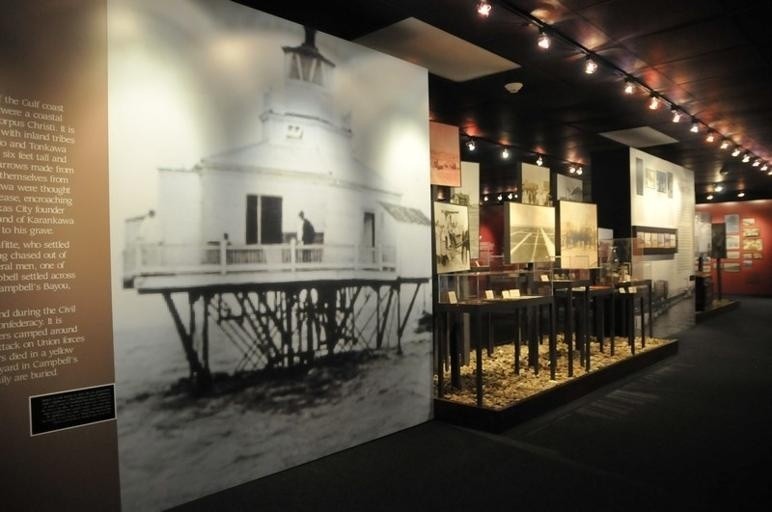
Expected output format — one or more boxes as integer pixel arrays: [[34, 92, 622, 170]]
[[297, 210, 314, 262], [138, 210, 162, 267]]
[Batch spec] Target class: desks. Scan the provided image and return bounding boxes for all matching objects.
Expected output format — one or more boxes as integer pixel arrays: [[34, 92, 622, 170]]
[[135, 276, 431, 387], [438, 277, 657, 410]]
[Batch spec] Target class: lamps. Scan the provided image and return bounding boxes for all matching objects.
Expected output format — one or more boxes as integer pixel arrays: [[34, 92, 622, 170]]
[[625, 79, 771, 176], [536, 24, 551, 50], [477, 0, 491, 17], [584, 52, 598, 75]]
